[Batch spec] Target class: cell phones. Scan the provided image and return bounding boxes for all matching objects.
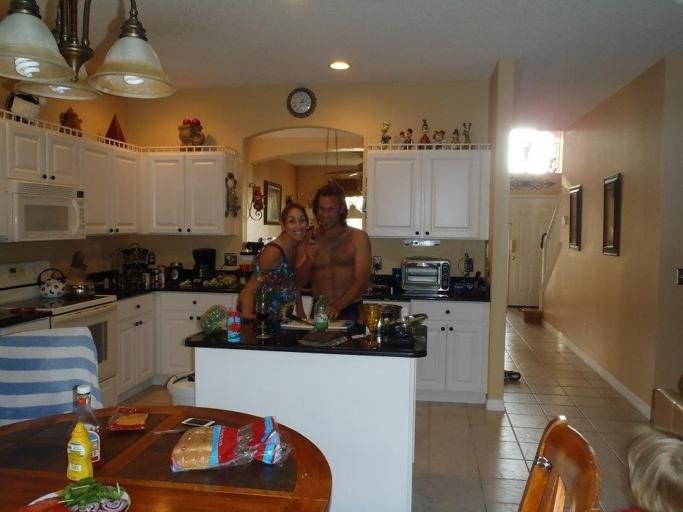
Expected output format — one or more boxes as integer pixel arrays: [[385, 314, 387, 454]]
[[181, 416, 215, 427]]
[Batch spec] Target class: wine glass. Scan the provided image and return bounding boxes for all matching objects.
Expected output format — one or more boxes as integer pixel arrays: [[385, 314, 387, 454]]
[[360, 303, 380, 346], [255, 299, 270, 339]]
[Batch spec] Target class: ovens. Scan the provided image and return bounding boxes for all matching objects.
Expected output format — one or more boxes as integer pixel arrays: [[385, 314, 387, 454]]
[[49, 300, 119, 384], [400, 258, 450, 293]]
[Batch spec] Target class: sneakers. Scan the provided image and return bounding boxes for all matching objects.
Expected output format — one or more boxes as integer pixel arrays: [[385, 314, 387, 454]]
[[505, 370, 521, 381]]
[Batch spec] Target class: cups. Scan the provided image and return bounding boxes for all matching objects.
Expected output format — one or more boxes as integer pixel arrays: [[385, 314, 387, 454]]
[[388, 267, 400, 295], [463, 283, 473, 293], [239, 263, 249, 278], [479, 285, 488, 297]]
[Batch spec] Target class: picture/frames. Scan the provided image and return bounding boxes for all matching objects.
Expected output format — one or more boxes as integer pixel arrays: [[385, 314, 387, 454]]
[[600, 172, 622, 257], [263, 180, 283, 226]]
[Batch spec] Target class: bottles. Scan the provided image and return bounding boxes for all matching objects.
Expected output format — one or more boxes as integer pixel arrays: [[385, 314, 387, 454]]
[[66, 420, 94, 482], [71, 385, 105, 469], [256, 250, 261, 270], [102, 246, 165, 290], [312, 294, 328, 331], [225, 310, 240, 342], [257, 285, 270, 303], [169, 262, 182, 280]]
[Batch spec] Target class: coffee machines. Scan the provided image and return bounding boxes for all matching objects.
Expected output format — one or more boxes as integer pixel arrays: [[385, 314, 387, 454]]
[[190, 248, 217, 281]]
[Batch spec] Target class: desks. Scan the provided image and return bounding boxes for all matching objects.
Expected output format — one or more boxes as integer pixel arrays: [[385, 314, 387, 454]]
[[1, 401, 335, 512]]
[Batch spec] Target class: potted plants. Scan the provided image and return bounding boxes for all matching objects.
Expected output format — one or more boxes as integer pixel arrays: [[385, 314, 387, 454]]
[[565, 184, 583, 252]]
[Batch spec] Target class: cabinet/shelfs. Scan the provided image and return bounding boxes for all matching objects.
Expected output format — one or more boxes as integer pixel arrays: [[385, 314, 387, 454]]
[[114, 290, 155, 405], [366, 148, 491, 242], [7, 119, 76, 187], [408, 298, 492, 407], [155, 291, 231, 386], [76, 139, 146, 236], [146, 150, 242, 238]]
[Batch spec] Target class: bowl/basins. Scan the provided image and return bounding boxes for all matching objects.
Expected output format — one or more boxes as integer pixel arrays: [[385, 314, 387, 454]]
[[199, 304, 226, 335]]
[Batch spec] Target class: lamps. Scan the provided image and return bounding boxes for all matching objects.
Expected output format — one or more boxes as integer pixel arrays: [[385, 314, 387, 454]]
[[248, 180, 264, 224], [1, 3, 189, 107]]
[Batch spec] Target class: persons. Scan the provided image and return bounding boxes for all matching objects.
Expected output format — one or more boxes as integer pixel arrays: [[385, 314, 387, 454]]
[[237, 203, 309, 320], [618, 427, 683, 512], [295, 185, 372, 320]]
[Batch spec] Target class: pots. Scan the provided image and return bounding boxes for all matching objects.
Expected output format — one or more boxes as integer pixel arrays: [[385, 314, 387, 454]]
[[61, 281, 96, 299]]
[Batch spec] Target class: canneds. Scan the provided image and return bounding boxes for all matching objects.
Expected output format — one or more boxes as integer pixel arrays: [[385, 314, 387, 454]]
[[141, 262, 183, 291]]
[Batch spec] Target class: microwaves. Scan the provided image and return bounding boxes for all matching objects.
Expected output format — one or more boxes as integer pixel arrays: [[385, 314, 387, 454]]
[[6, 178, 87, 240]]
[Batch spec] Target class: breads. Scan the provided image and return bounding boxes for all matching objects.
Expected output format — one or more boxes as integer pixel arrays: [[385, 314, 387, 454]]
[[173, 427, 213, 470]]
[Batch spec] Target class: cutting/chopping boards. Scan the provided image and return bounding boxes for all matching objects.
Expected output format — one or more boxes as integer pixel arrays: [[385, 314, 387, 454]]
[[281, 317, 354, 330]]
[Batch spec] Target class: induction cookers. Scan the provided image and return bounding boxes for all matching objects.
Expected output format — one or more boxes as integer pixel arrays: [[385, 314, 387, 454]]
[[0, 293, 117, 318]]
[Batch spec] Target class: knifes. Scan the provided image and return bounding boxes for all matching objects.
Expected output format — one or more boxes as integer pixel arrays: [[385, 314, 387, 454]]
[[288, 313, 315, 327]]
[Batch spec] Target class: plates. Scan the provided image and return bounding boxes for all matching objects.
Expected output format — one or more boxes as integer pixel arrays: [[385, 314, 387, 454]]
[[29, 487, 130, 512]]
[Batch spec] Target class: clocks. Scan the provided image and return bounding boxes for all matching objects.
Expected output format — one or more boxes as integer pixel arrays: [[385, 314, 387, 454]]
[[284, 87, 316, 118]]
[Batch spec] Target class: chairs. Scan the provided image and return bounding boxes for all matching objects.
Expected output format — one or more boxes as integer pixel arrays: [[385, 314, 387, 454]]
[[511, 415, 603, 512], [0, 322, 102, 428]]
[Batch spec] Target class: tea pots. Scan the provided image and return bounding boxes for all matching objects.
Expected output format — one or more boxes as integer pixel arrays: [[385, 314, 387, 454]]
[[36, 266, 68, 299]]
